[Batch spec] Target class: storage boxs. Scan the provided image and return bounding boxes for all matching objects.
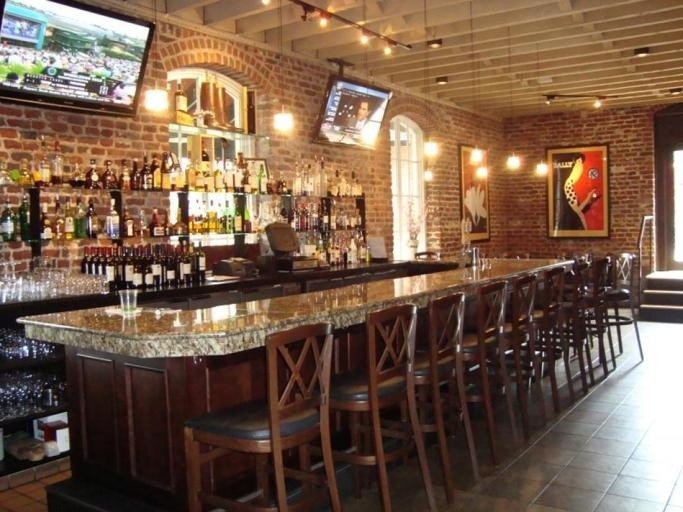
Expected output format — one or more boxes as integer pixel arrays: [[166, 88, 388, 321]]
[[31, 412, 70, 454]]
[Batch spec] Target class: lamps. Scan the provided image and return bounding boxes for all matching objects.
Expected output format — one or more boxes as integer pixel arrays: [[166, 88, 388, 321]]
[[544, 92, 617, 110], [143, 0, 171, 116], [408, 0, 552, 182], [258, 0, 412, 55], [0, 181, 369, 281], [266, 0, 296, 135], [632, 46, 649, 59], [668, 85, 682, 96]]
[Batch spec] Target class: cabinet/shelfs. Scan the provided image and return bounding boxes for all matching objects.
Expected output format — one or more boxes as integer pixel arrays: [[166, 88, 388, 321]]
[[167, 126, 256, 176], [0, 295, 113, 481]]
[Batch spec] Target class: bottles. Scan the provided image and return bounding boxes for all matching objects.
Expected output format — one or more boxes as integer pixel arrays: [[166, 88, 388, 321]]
[[174, 81, 187, 112], [0, 194, 121, 243], [17, 136, 363, 197], [82, 242, 205, 288], [123, 198, 361, 238], [471, 247, 479, 260], [297, 233, 372, 263]]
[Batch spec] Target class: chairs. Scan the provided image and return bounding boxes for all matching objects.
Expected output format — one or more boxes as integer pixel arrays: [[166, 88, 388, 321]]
[[182, 321, 343, 512]]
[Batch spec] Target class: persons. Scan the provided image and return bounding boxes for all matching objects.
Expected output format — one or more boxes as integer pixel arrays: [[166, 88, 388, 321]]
[[1, 42, 142, 83], [352, 99, 370, 130]]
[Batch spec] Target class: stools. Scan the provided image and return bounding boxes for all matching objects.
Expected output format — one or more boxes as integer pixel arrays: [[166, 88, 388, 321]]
[[296, 303, 439, 512], [418, 249, 644, 469], [363, 291, 481, 502]]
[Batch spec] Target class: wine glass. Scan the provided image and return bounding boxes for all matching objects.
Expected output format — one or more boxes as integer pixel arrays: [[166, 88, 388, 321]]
[[0, 256, 106, 304]]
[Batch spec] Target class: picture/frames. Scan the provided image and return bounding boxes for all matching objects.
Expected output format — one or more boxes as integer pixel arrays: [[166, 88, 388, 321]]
[[543, 142, 610, 241], [458, 143, 491, 244]]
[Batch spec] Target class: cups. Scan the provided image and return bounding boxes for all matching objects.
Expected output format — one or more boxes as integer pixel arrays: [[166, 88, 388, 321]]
[[118, 288, 138, 312], [0, 368, 69, 417], [477, 258, 492, 271], [0, 326, 53, 360]]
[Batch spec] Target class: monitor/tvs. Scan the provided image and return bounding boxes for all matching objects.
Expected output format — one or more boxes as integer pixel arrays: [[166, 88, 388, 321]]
[[0, 0, 155, 117], [312, 73, 392, 151], [266, 222, 300, 257]]
